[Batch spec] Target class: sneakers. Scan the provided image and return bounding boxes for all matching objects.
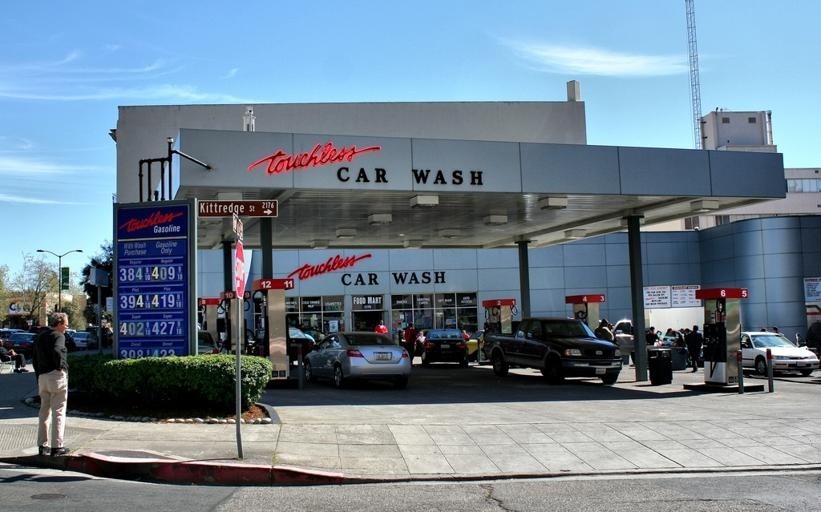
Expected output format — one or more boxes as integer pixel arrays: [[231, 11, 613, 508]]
[[51, 447, 70, 457], [39, 445, 52, 456]]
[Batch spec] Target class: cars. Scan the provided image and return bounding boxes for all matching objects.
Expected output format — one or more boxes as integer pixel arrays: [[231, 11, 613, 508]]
[[198, 331, 218, 354], [66, 326, 113, 351], [247, 327, 314, 362], [672, 330, 705, 366], [415, 329, 431, 355], [422, 329, 469, 366], [466, 330, 487, 361], [2, 333, 38, 356], [305, 331, 412, 388], [740, 331, 819, 376]]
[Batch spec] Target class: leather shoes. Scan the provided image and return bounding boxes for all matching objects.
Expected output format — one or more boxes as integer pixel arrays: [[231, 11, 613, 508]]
[[14, 369, 22, 373], [20, 369, 28, 372]]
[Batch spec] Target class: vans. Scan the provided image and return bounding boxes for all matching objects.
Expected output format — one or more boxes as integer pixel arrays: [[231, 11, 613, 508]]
[[568, 318, 636, 359], [1, 328, 24, 342], [198, 323, 224, 349]]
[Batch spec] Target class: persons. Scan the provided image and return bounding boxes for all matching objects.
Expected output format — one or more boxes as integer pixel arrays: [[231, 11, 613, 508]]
[[405, 321, 422, 362], [462, 329, 471, 339], [795, 333, 805, 347], [0, 339, 29, 374], [374, 319, 390, 336], [593, 318, 704, 374], [33, 313, 70, 458], [773, 327, 784, 336], [417, 330, 426, 344], [400, 329, 409, 342]]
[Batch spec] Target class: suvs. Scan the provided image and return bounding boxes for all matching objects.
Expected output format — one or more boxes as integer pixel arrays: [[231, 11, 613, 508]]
[[30, 326, 69, 348]]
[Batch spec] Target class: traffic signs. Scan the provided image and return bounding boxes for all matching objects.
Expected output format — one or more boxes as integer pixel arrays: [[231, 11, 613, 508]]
[[196, 199, 278, 218]]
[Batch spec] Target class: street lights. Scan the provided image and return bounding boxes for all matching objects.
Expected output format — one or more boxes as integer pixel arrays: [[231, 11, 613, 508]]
[[37, 249, 84, 312]]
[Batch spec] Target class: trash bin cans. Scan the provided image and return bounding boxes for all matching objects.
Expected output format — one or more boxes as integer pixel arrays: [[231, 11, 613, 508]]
[[670, 347, 688, 370], [647, 348, 672, 384]]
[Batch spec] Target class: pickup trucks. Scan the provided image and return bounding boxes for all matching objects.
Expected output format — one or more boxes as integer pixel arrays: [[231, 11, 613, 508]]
[[487, 317, 623, 385]]
